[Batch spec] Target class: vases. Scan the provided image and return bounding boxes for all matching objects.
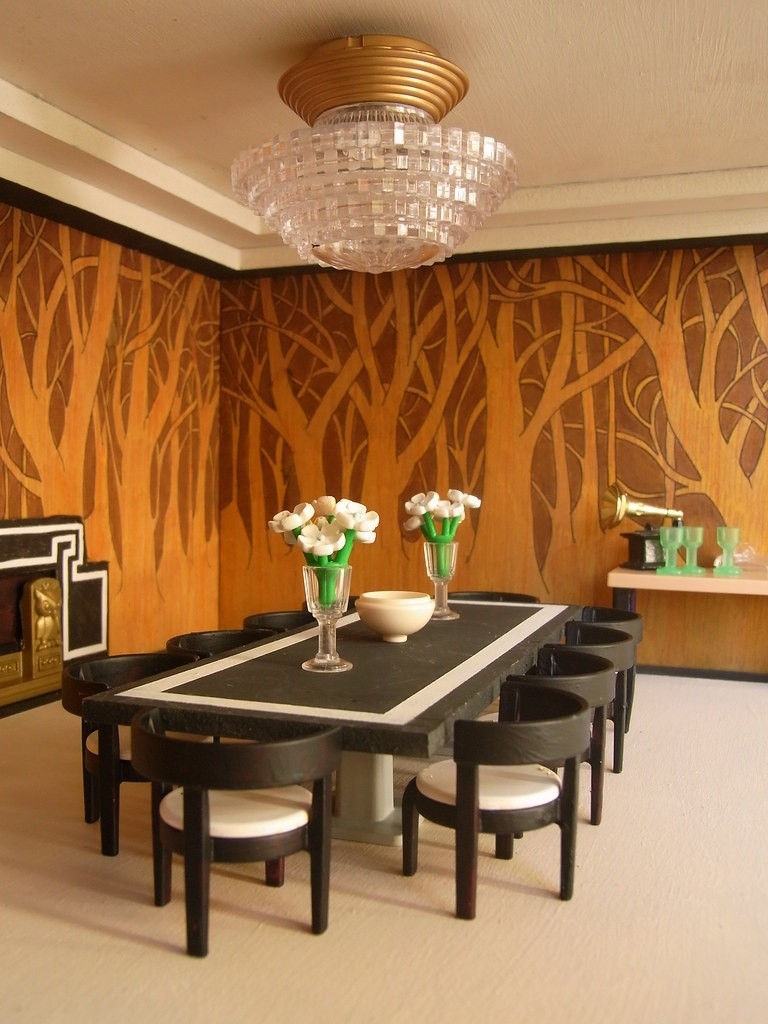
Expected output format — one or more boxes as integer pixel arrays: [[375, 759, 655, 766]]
[[423, 542, 461, 621], [301, 564, 353, 673]]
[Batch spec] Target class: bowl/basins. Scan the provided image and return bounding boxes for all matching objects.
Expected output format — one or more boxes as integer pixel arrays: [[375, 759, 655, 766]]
[[355, 591, 435, 643]]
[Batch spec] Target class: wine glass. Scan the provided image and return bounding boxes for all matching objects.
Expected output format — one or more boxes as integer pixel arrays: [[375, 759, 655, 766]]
[[656, 526, 707, 576], [713, 527, 741, 575], [301, 565, 352, 672], [424, 542, 460, 620]]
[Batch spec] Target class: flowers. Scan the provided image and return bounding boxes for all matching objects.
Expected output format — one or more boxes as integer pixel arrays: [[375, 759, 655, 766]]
[[264, 495, 379, 605], [402, 488, 482, 581]]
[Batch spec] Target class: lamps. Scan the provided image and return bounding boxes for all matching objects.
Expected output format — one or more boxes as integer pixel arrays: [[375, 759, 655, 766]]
[[234, 32, 522, 274]]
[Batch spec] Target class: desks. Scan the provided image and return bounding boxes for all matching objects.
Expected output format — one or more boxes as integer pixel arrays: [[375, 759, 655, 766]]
[[605, 566, 768, 684], [81, 599, 587, 848]]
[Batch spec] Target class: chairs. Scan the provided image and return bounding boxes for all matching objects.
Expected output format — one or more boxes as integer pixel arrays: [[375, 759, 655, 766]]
[[59, 592, 643, 958]]
